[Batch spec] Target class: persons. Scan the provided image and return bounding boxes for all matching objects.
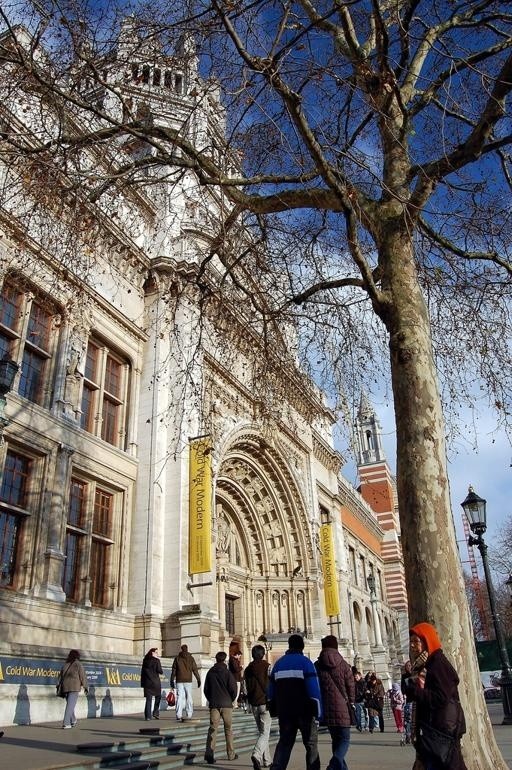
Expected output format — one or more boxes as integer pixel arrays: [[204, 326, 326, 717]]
[[268, 634, 321, 770], [141, 648, 164, 721], [240, 645, 271, 770], [202, 651, 238, 764], [57, 649, 89, 729], [170, 645, 201, 720], [314, 635, 355, 769], [348, 660, 412, 745], [229, 650, 244, 709], [409, 623, 468, 770]]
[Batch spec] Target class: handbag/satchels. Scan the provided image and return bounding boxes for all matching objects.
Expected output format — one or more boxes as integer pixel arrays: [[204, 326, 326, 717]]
[[56, 683, 66, 698], [415, 725, 456, 769], [167, 692, 175, 706]]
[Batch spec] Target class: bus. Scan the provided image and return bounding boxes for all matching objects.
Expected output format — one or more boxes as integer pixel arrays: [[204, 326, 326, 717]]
[[476, 637, 512, 699]]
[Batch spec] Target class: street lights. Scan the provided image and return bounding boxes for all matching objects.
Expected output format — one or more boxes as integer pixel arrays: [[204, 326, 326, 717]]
[[460, 485, 512, 725]]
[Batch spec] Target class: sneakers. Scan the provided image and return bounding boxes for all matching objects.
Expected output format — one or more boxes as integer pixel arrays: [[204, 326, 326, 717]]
[[251, 756, 272, 770], [204, 754, 238, 764], [62, 719, 77, 729], [177, 715, 191, 722], [146, 714, 159, 720], [356, 724, 384, 734]]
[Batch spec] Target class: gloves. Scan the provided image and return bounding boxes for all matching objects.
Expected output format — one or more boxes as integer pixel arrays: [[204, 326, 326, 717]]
[[85, 688, 88, 695], [405, 680, 416, 698], [170, 682, 174, 688], [197, 680, 201, 688]]
